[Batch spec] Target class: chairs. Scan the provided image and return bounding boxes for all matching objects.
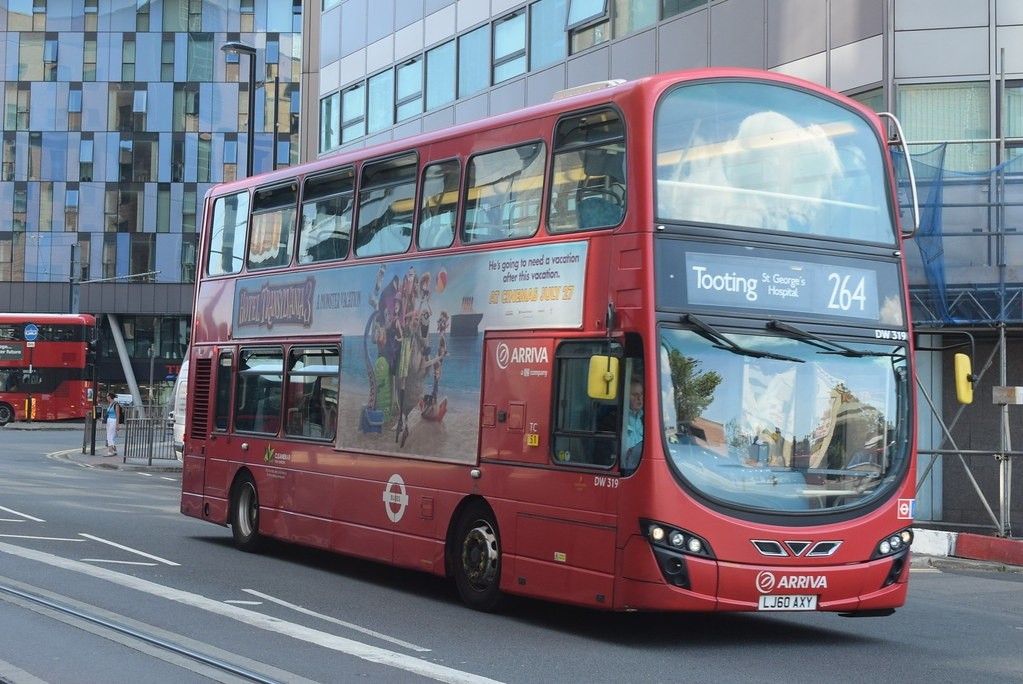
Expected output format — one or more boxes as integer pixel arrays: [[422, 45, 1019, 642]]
[[314, 186, 625, 260]]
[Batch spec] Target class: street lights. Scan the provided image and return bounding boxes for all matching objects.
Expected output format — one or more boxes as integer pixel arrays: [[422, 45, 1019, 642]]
[[220, 41, 256, 175]]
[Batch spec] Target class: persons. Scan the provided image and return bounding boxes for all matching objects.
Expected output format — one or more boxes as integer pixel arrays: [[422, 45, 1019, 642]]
[[103, 391, 120, 457], [623, 378, 644, 468]]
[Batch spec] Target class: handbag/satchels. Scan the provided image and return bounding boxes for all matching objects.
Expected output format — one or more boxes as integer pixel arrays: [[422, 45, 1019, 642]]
[[114, 402, 124, 425]]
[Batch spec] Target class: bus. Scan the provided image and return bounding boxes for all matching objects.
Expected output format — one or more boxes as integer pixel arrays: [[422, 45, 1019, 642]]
[[178, 64, 977, 619], [0, 310, 98, 428]]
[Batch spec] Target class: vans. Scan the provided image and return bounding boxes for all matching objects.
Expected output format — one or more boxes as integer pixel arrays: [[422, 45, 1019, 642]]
[[172, 358, 304, 463]]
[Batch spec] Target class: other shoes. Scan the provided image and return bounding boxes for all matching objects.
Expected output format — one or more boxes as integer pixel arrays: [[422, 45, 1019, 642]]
[[108, 450, 117, 455]]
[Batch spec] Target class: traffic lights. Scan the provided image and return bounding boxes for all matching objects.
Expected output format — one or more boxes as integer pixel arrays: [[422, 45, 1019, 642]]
[[87, 338, 99, 369]]
